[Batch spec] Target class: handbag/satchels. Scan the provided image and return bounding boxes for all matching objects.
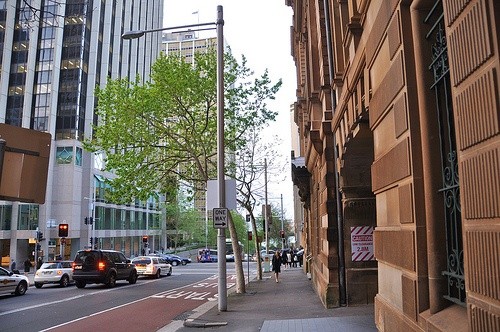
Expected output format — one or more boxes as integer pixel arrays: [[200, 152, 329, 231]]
[[293, 256, 297, 261]]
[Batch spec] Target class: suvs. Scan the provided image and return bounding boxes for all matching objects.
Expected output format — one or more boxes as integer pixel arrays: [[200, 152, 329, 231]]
[[72, 249, 137, 289]]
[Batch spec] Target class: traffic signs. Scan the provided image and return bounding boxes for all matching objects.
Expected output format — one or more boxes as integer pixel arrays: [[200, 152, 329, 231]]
[[213, 207, 228, 229]]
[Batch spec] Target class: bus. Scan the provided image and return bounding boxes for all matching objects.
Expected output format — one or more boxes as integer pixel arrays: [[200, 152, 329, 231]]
[[225, 238, 244, 261], [198, 249, 218, 262]]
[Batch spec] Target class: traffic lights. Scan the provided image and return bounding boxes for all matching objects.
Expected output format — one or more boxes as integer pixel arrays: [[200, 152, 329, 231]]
[[89, 237, 93, 244], [38, 231, 46, 243], [90, 217, 93, 224], [84, 217, 89, 225], [282, 231, 285, 238], [95, 237, 98, 244], [58, 223, 69, 237]]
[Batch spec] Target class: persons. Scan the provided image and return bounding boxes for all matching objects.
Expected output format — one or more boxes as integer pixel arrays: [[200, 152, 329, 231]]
[[282, 251, 288, 268], [143, 249, 145, 256], [24, 257, 32, 272], [289, 251, 302, 268], [272, 251, 281, 282], [11, 259, 16, 272], [37, 257, 43, 270]]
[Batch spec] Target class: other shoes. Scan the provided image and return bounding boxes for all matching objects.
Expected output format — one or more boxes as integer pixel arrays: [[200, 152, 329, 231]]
[[276, 279, 278, 283]]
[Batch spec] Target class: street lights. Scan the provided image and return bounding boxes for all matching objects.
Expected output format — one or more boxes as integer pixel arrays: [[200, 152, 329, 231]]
[[122, 4, 228, 312]]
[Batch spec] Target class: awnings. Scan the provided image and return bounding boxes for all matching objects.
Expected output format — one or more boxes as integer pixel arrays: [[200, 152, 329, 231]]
[[120, 184, 124, 188], [94, 175, 105, 181], [154, 191, 161, 197], [136, 187, 141, 191], [107, 178, 113, 182]]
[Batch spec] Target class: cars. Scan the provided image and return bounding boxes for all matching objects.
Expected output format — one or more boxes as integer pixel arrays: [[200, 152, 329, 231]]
[[152, 254, 192, 266], [261, 250, 276, 260], [34, 261, 75, 288], [296, 249, 305, 261], [244, 254, 257, 262], [131, 256, 172, 278], [0, 267, 30, 296]]
